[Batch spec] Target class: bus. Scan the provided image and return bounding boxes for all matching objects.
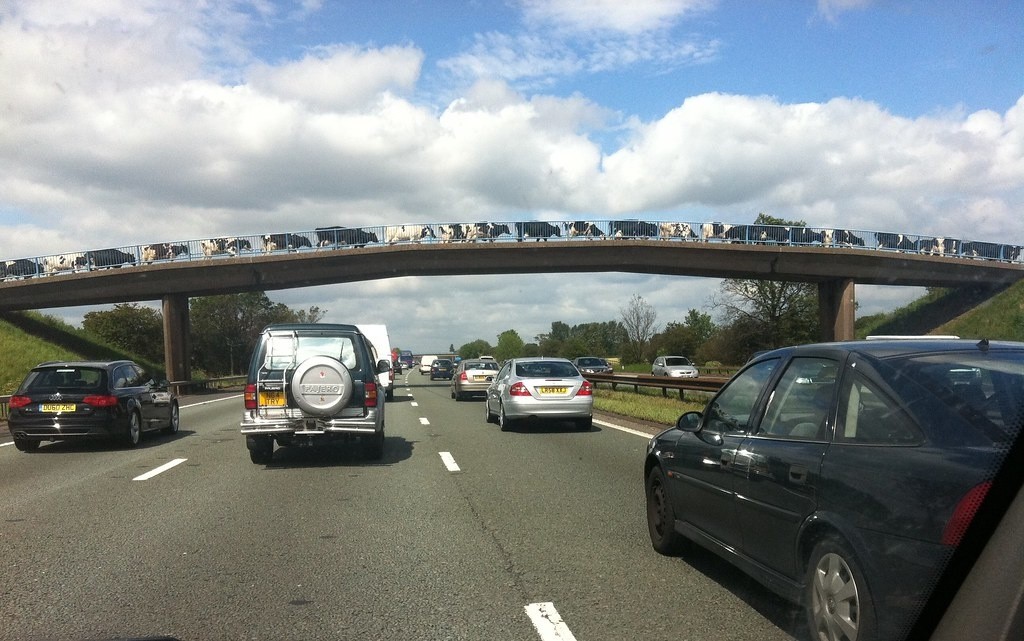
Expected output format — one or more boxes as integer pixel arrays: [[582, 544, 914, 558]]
[[400, 350, 413, 368]]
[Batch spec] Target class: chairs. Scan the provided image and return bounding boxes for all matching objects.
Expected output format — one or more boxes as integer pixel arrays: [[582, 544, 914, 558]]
[[515, 365, 526, 376], [791, 384, 886, 439], [549, 366, 568, 377], [65, 369, 82, 386]]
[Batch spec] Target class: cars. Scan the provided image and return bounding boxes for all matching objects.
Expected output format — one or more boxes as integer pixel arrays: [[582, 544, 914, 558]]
[[950, 366, 982, 390], [644, 338, 1024, 641], [651, 356, 699, 378], [412, 362, 416, 367], [478, 356, 498, 369], [745, 349, 804, 383], [485, 357, 593, 432], [393, 364, 402, 375], [430, 359, 454, 380], [400, 361, 409, 370], [599, 358, 614, 373], [571, 357, 609, 374], [449, 359, 501, 401]]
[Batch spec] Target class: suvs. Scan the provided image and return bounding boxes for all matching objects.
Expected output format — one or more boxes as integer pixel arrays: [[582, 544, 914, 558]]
[[239, 323, 389, 464], [7, 361, 180, 452]]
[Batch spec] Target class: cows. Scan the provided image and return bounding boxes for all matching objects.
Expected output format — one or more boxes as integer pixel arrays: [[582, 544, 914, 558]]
[[384, 223, 436, 246], [84, 249, 136, 271], [439, 221, 512, 244], [700, 225, 865, 248], [562, 221, 606, 241], [199, 236, 254, 260], [315, 226, 379, 249], [260, 232, 312, 256], [608, 219, 661, 240], [40, 251, 88, 277], [515, 221, 562, 242], [141, 243, 188, 265], [0, 259, 44, 283], [655, 221, 700, 241], [874, 232, 1020, 264]]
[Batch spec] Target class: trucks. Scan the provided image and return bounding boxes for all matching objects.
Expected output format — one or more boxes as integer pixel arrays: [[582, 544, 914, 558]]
[[418, 356, 438, 375], [356, 324, 396, 399]]
